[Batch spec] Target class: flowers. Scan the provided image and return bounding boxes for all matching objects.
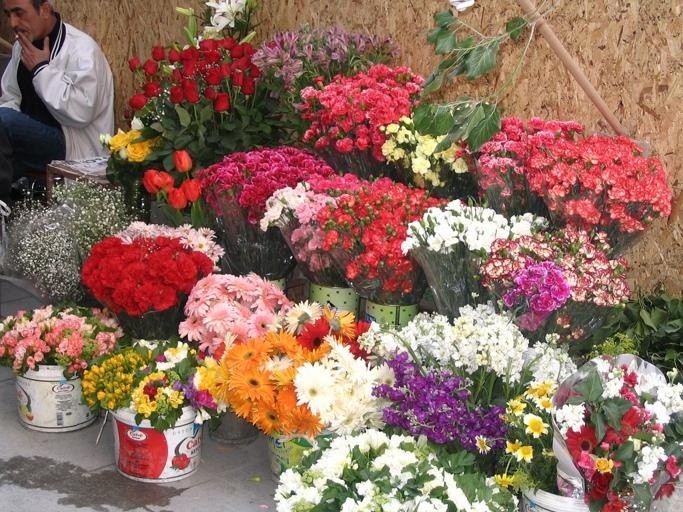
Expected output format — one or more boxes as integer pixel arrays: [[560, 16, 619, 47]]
[[0, 0, 683, 512]]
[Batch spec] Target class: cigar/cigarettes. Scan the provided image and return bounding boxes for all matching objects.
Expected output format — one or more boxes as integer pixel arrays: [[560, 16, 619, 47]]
[[12, 34, 19, 40]]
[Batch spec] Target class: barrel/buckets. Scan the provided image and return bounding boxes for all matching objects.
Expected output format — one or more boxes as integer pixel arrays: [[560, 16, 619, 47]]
[[15, 365, 98, 432], [519, 485, 596, 512], [109, 405, 202, 483]]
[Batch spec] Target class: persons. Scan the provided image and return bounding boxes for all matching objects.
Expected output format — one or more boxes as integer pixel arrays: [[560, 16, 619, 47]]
[[0, 0, 115, 212]]
